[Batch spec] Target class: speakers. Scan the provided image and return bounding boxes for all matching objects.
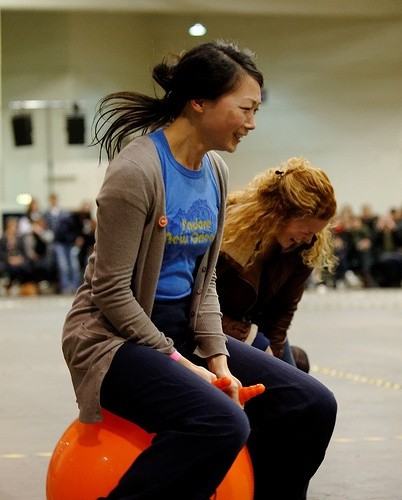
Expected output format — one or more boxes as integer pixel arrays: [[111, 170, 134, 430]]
[[67, 116, 84, 144], [13, 114, 31, 146]]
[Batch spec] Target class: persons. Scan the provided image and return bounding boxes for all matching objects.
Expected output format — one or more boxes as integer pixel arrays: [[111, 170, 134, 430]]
[[62, 41, 337, 500], [303, 202, 402, 295], [0, 191, 98, 296], [215, 157, 339, 374]]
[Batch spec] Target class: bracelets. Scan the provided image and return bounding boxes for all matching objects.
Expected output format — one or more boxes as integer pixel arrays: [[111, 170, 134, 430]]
[[169, 351, 182, 361]]
